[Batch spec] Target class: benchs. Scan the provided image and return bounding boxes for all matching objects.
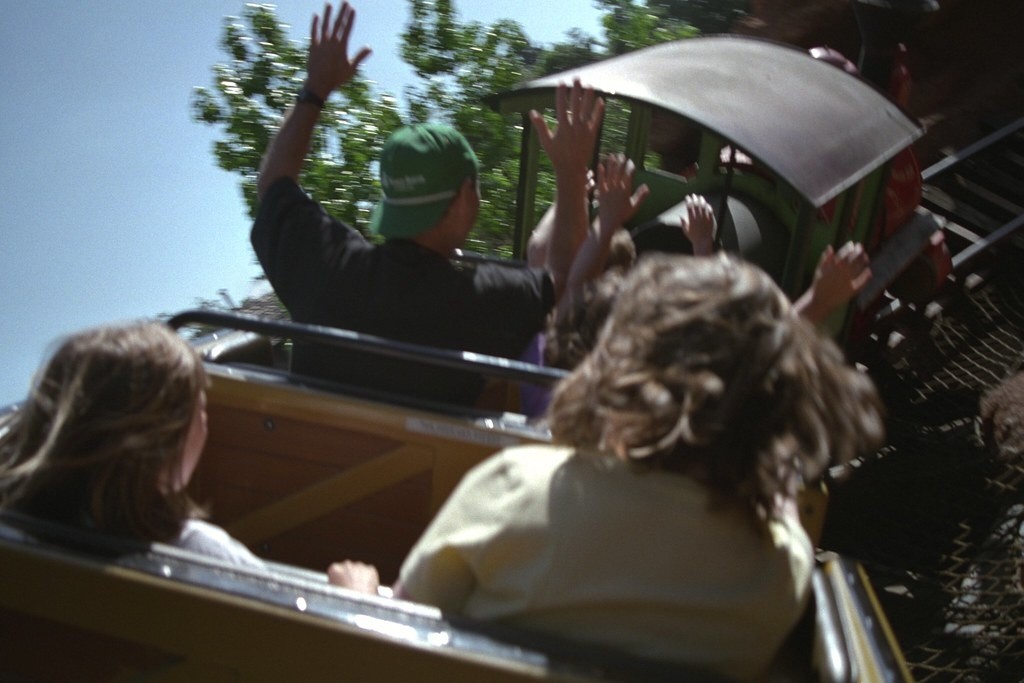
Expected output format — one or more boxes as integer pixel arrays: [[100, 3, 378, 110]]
[[0, 507, 870, 683], [169, 308, 573, 586]]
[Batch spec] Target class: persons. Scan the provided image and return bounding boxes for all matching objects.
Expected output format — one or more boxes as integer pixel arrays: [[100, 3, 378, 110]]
[[321, 251, 882, 675], [522, 151, 874, 420], [0, 318, 271, 573], [244, 0, 607, 405]]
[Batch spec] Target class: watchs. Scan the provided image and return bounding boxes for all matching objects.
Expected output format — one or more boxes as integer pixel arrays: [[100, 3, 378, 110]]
[[296, 88, 325, 109]]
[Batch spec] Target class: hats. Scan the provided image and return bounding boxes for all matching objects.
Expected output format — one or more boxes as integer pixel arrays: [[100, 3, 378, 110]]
[[368, 122, 479, 238]]
